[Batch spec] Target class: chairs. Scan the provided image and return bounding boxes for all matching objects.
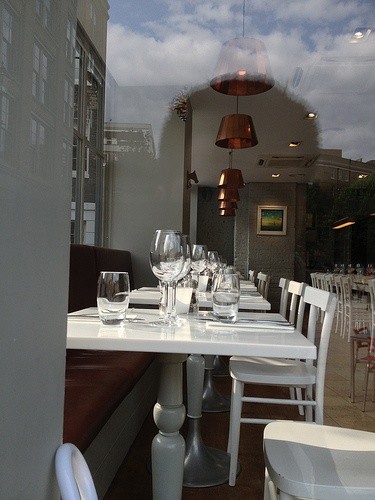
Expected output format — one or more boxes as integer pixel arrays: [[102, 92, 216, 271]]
[[55, 443, 99, 500], [223, 270, 375, 500]]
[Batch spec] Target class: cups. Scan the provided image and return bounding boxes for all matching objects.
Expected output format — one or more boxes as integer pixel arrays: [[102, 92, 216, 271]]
[[97, 271, 130, 324], [206, 251, 240, 322], [332, 264, 375, 276]]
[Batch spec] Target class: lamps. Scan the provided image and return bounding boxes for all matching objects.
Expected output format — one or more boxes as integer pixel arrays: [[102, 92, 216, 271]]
[[350, 26, 371, 44], [187, 170, 200, 189], [358, 158, 367, 179], [330, 159, 357, 230], [210, 0, 318, 216]]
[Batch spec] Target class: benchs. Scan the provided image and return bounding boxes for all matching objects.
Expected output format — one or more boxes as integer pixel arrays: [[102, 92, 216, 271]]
[[63, 244, 160, 500]]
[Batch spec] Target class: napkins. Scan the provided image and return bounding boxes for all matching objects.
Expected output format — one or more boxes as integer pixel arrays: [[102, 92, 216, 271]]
[[68, 314, 137, 323], [206, 320, 296, 330]]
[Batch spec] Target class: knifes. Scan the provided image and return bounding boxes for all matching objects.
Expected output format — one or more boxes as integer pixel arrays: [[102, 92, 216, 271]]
[[198, 318, 293, 326], [66, 314, 145, 321]]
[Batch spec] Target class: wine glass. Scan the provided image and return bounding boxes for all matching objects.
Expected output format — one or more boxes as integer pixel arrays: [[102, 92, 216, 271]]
[[148, 229, 207, 328]]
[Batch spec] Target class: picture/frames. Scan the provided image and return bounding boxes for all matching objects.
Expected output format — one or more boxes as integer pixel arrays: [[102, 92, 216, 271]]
[[257, 205, 287, 236]]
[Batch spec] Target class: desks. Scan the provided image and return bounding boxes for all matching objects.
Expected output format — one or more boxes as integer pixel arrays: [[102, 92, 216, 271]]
[[329, 274, 375, 292], [66, 280, 317, 500]]
